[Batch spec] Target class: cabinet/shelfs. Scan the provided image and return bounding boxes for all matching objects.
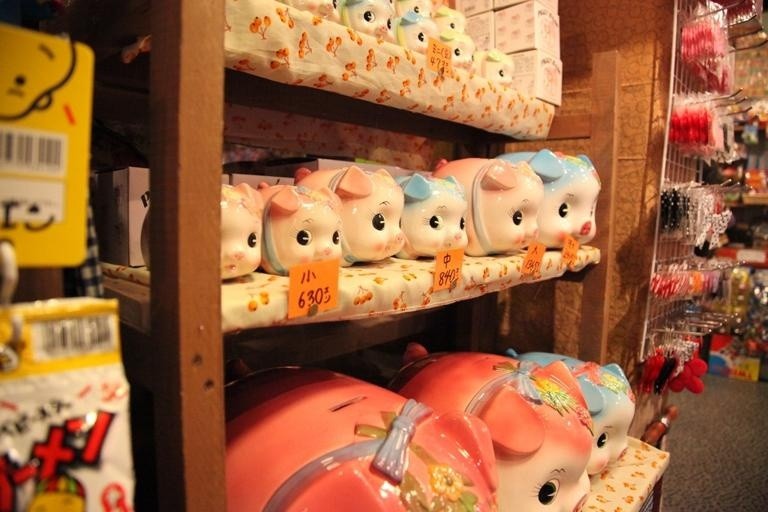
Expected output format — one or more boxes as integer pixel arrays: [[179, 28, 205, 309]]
[[96, 0, 672, 512]]
